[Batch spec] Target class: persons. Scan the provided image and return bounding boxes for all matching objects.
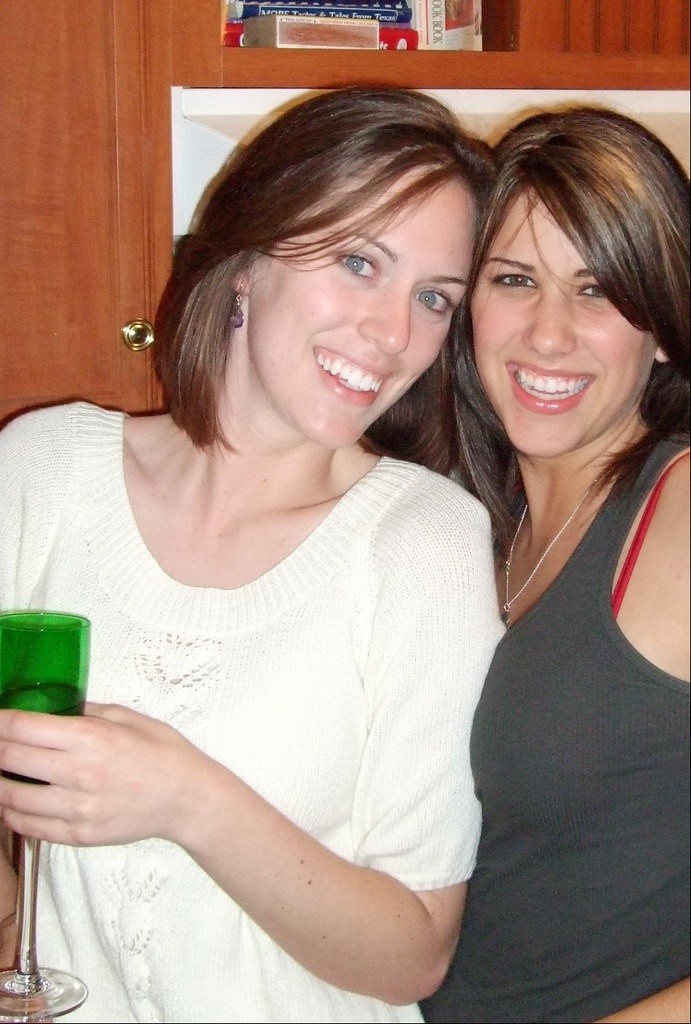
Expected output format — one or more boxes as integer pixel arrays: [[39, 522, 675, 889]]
[[0, 88, 497, 1023], [418, 107, 691, 1023]]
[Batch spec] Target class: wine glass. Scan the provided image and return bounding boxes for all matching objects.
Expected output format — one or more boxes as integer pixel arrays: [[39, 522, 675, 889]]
[[0, 611, 96, 1022]]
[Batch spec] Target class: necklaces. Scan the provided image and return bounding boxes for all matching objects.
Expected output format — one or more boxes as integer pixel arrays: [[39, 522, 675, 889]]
[[502, 479, 597, 623]]
